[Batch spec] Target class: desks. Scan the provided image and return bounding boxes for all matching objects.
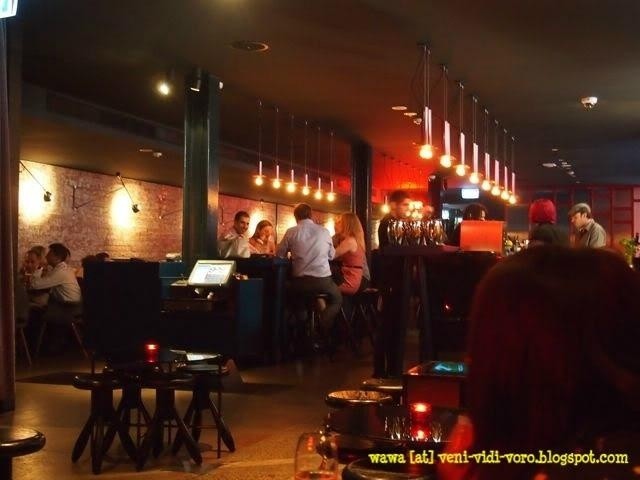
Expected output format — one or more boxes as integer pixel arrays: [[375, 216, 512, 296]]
[[385, 245, 459, 370]]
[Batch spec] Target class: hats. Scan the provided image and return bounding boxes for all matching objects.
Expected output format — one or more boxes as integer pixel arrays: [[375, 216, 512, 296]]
[[568, 203, 591, 217], [529, 198, 556, 223]]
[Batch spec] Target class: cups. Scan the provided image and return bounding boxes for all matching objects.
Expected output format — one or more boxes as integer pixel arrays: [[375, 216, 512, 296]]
[[385, 219, 449, 248], [295, 430, 344, 479]]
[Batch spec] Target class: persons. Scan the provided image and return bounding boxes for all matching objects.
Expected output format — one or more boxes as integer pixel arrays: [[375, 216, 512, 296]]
[[370, 190, 415, 379], [444, 202, 486, 247], [527, 199, 568, 249], [19, 243, 83, 360], [566, 203, 606, 250], [217, 204, 370, 353], [465, 246, 640, 480]]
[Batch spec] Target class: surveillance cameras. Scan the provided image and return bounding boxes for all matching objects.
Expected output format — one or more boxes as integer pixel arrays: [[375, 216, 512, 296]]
[[580, 96, 598, 110]]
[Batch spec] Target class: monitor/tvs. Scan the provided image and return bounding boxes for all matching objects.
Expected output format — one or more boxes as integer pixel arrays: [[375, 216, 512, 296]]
[[188, 260, 236, 286]]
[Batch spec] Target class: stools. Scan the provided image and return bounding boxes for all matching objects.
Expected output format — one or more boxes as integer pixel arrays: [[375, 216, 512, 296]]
[[290, 291, 384, 360], [1, 426, 46, 480], [72, 353, 234, 474], [15, 323, 34, 368], [320, 379, 436, 480], [36, 317, 90, 360]]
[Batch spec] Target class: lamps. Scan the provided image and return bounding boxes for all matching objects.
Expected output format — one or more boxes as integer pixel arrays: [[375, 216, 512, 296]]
[[255, 97, 336, 201], [420, 44, 518, 205], [190, 68, 202, 92]]
